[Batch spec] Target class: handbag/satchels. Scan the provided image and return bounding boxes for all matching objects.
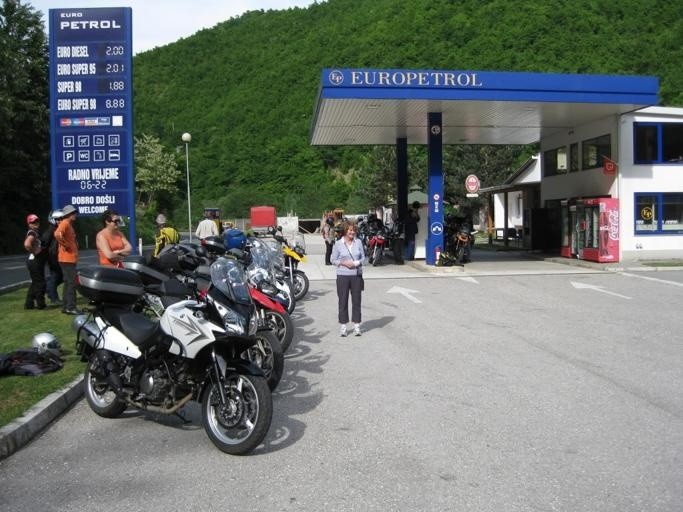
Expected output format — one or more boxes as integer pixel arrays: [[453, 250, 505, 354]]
[[360, 277, 365, 290]]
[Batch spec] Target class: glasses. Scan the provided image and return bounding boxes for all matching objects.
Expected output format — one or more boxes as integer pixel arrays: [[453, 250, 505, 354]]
[[107, 218, 121, 224], [31, 220, 39, 223]]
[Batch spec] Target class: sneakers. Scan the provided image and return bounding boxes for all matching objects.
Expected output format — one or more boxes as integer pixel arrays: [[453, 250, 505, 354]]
[[352, 325, 362, 336], [23, 299, 84, 316], [339, 327, 349, 337]]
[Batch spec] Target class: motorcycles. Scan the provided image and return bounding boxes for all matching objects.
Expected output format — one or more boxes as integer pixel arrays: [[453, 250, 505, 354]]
[[440, 212, 479, 267], [349, 215, 390, 268]]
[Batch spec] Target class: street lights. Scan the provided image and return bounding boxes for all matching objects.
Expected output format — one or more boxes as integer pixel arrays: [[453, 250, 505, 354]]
[[181, 132, 193, 243]]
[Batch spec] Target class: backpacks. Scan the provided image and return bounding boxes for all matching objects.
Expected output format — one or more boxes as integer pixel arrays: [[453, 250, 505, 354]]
[[1, 347, 65, 378]]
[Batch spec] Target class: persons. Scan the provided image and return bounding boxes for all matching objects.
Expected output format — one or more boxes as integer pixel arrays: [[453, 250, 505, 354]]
[[210, 208, 223, 234], [20, 212, 49, 312], [274, 225, 283, 238], [329, 219, 368, 337], [94, 208, 133, 269], [321, 200, 421, 266], [151, 212, 179, 263], [194, 210, 220, 242], [53, 202, 82, 316], [39, 208, 65, 307]]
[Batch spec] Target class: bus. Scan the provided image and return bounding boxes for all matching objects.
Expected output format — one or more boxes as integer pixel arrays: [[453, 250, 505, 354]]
[[204, 206, 223, 236]]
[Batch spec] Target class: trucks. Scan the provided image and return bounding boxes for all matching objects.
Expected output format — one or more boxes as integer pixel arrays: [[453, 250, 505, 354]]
[[248, 205, 276, 237]]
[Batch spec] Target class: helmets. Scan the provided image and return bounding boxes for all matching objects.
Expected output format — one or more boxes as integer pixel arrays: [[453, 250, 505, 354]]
[[31, 332, 63, 356], [156, 213, 168, 226], [48, 209, 64, 225]]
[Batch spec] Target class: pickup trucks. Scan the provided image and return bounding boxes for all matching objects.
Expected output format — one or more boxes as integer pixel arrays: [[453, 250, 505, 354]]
[[222, 221, 232, 230]]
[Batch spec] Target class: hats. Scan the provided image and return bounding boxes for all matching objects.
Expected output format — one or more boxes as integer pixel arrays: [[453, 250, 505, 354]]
[[61, 205, 78, 216], [26, 214, 40, 224]]
